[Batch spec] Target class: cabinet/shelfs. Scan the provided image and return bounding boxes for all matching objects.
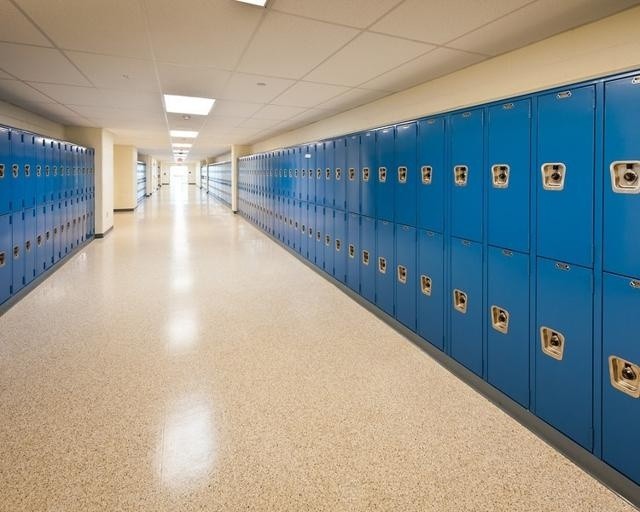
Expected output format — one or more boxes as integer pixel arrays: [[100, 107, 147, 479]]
[[0, 123, 95, 316], [198, 159, 232, 204], [136, 159, 147, 206]]
